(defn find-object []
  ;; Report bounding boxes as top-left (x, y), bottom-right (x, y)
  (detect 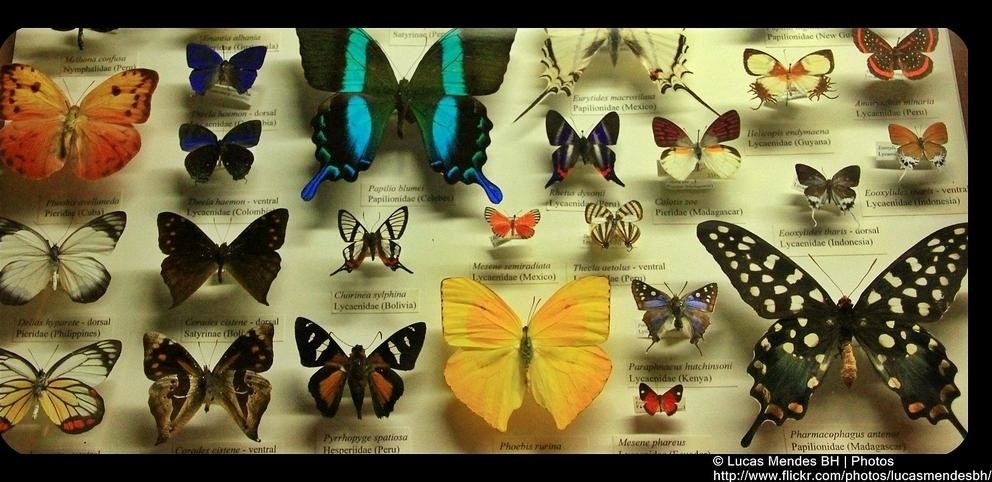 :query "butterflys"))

top-left (0, 29), bottom-right (970, 452)
